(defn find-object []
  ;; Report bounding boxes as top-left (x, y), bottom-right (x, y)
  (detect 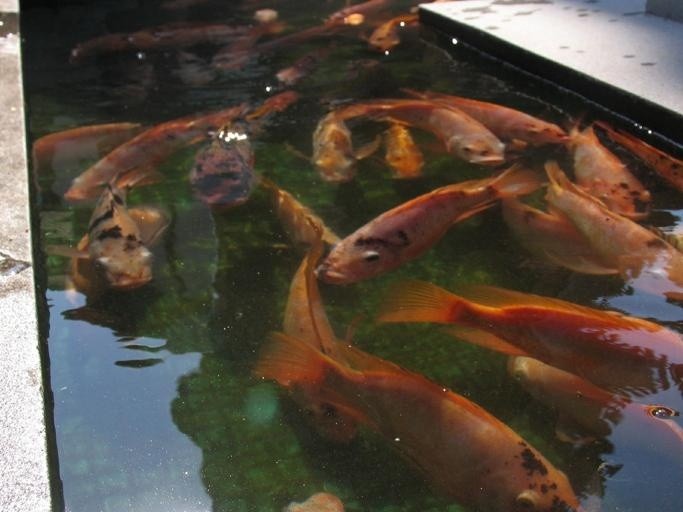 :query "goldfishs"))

top-left (70, 1), bottom-right (422, 88)
top-left (33, 89), bottom-right (683, 304)
